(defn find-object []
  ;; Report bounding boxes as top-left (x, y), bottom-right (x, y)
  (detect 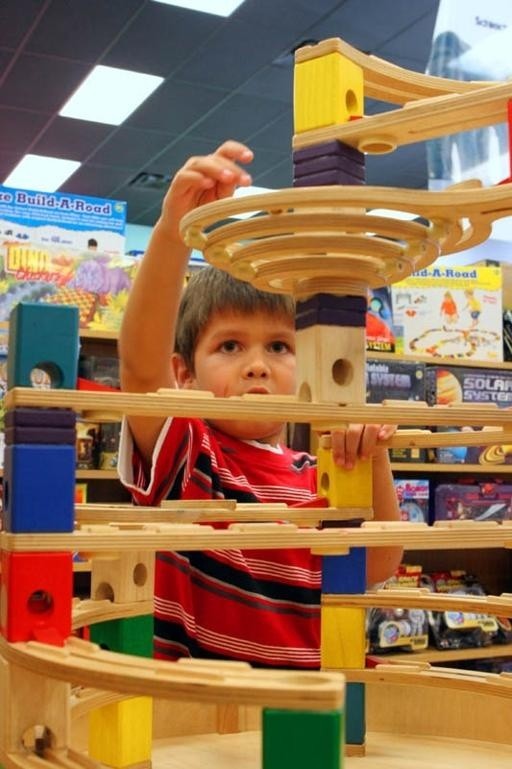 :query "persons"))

top-left (118, 143), bottom-right (403, 674)
